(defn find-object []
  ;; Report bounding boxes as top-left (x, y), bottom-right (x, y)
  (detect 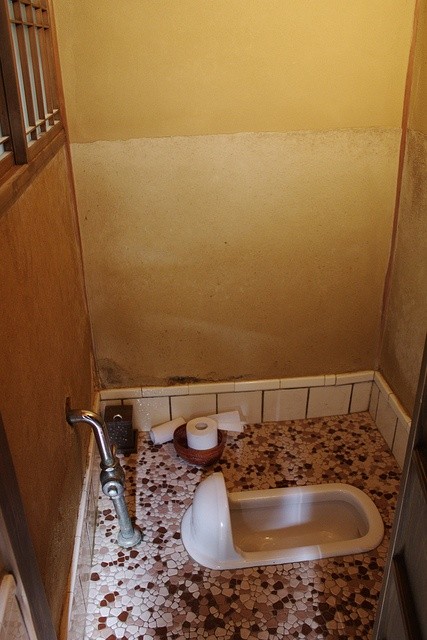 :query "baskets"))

top-left (173, 422), bottom-right (227, 466)
